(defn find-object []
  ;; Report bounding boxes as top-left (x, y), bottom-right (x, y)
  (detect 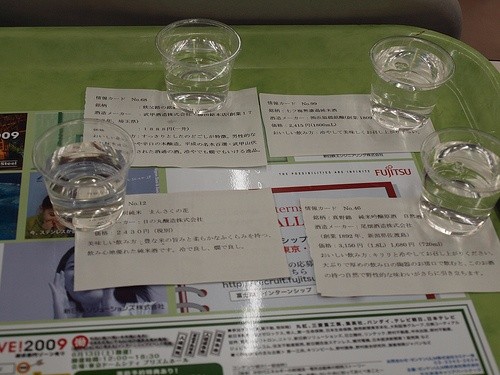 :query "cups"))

top-left (419, 128), bottom-right (500, 237)
top-left (156, 18), bottom-right (242, 114)
top-left (32, 118), bottom-right (136, 231)
top-left (370, 36), bottom-right (456, 131)
